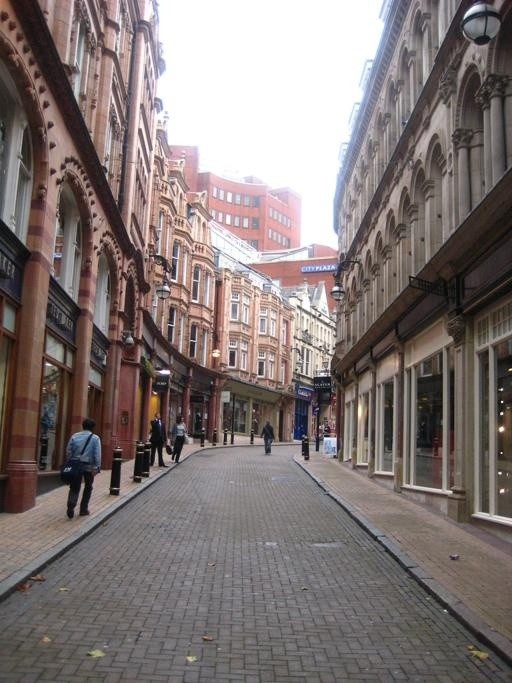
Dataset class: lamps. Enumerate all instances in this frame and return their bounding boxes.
[457,0,503,47]
[211,334,221,357]
[153,265,173,299]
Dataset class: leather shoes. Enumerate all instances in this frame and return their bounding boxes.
[158,464,170,467]
[67,509,90,518]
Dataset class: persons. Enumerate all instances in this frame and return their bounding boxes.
[260,419,276,454]
[317,418,336,439]
[64,417,102,517]
[171,415,191,462]
[150,411,169,467]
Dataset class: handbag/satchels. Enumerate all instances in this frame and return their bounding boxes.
[166,445,172,455]
[61,460,81,484]
